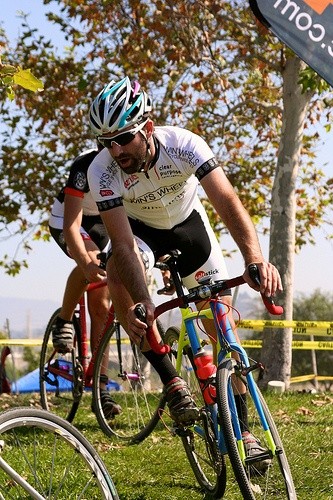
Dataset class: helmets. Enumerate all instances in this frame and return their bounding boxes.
[88,75,152,137]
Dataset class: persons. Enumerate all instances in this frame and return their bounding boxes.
[49,141,126,425]
[95,75,283,478]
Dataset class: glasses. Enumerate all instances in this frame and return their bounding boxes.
[104,116,149,150]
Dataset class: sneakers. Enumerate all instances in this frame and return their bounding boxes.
[91,390,125,415]
[51,317,75,354]
[242,435,271,470]
[161,382,200,422]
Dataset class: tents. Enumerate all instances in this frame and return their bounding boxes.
[7,358,121,392]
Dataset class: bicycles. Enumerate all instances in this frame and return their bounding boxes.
[0,406,120,500]
[38,252,175,446]
[133,247,298,500]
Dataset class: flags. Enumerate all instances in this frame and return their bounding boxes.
[248,0,333,90]
[0,346,12,394]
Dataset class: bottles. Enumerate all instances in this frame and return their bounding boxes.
[194,340,217,404]
